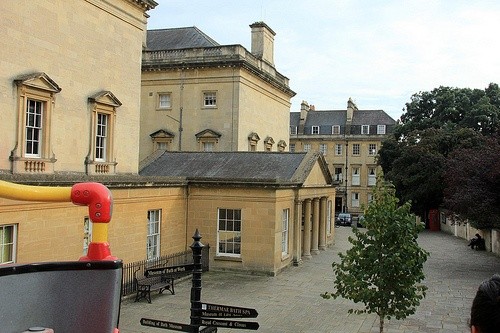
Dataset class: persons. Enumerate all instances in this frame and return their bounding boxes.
[468,232,482,248]
[468,274,500,333]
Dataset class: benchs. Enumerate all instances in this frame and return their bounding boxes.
[134,274,175,304]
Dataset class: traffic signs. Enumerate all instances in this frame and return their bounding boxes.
[139,264,260,333]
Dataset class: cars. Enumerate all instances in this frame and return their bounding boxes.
[357,215,367,228]
[336,214,352,226]
